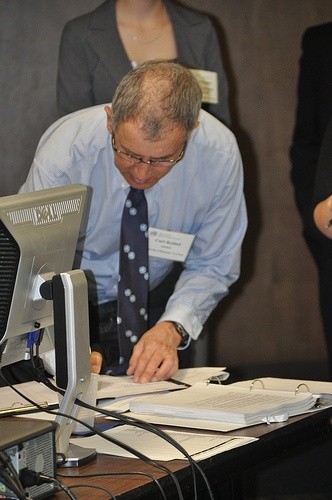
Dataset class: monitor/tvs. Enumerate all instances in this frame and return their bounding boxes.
[0,184,99,468]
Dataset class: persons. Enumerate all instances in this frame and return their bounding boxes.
[15,59,250,385]
[56,0,235,371]
[289,20,332,381]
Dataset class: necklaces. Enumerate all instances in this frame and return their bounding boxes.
[116,12,166,45]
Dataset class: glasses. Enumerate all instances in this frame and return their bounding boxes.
[111,130,188,168]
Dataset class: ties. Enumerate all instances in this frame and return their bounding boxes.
[102,185,150,376]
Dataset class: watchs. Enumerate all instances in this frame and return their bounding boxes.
[172,321,190,346]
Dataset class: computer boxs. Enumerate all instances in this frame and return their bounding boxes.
[0,417,56,500]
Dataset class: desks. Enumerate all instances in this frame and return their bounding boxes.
[14,407,332,500]
